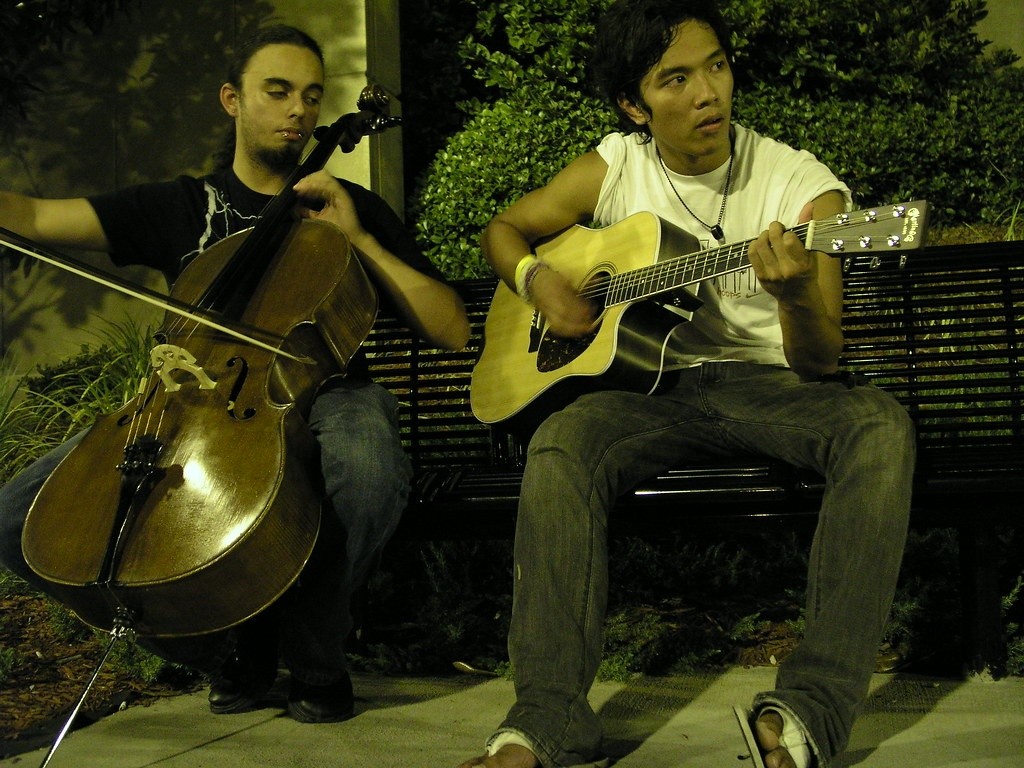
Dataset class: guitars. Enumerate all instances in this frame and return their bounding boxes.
[471,198,931,429]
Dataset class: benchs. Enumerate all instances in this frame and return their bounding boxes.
[362,239,1024,671]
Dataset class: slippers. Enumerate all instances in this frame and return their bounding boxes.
[484,728,537,758]
[732,700,814,768]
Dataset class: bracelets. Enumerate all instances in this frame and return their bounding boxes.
[514,254,548,302]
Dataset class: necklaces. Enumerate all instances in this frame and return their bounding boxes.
[656,142,734,240]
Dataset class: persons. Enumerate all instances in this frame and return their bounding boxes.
[457,0,916,768]
[0,21,471,724]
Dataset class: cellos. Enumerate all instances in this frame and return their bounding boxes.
[6,82,407,768]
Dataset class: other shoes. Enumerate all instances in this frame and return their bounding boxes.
[286,690,339,723]
[208,681,255,714]
[454,653,499,675]
